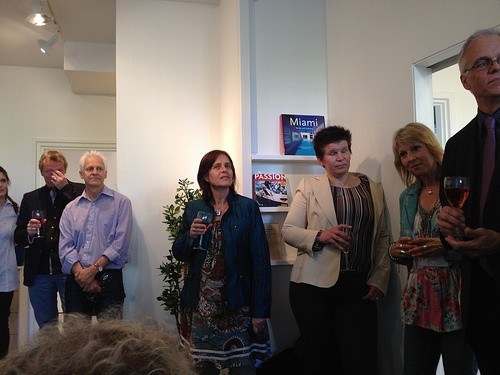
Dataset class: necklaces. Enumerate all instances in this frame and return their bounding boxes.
[328,172,349,187]
[213,204,228,216]
[423,183,436,195]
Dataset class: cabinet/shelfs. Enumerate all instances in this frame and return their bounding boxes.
[251,155,319,266]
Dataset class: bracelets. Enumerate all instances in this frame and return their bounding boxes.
[389,242,400,261]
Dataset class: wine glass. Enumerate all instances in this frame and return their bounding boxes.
[193,211,212,251]
[444,176,470,236]
[32,209,44,239]
[84,271,112,303]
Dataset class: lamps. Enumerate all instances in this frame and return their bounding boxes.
[24,0,54,27]
[37,33,59,56]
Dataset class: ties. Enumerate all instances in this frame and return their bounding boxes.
[479,116,496,227]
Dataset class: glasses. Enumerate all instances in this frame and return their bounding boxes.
[462,56,500,73]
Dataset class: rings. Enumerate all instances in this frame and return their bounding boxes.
[401,250,405,254]
[423,245,428,249]
[400,243,402,247]
[263,326,265,328]
[54,177,57,180]
[376,297,379,301]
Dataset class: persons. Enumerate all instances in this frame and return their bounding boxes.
[437,29,500,375]
[281,125,391,375]
[0,314,195,375]
[265,179,287,194]
[0,166,20,360]
[171,150,272,375]
[388,122,478,375]
[58,150,132,321]
[13,150,85,328]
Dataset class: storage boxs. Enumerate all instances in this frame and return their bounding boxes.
[280,114,325,155]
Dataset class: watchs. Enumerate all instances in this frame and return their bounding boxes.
[93,262,103,272]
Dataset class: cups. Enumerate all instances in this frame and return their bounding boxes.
[404,228,432,256]
[335,228,350,251]
